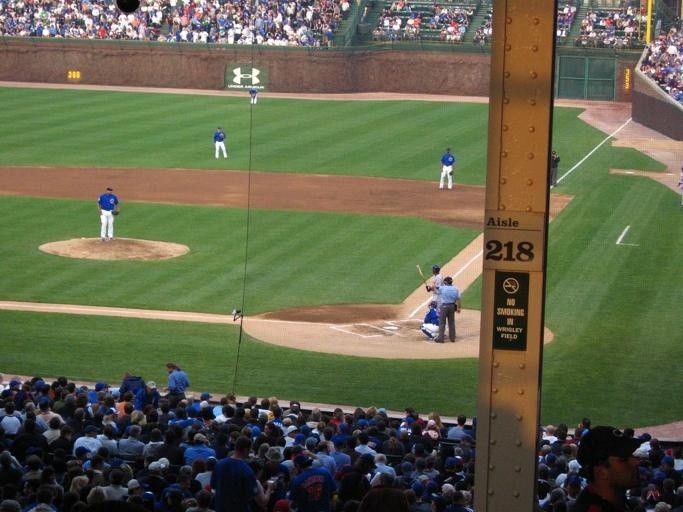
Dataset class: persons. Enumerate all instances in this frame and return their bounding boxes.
[97,186,120,242]
[551,150,560,186]
[437,147,453,191]
[1,0,683,111]
[0,360,683,512]
[213,126,228,160]
[418,265,461,343]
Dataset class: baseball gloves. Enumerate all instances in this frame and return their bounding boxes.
[219,133,225,138]
[112,210,118,215]
[450,171,453,175]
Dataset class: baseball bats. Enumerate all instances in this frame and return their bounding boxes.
[416,265,431,293]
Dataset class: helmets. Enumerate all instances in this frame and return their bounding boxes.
[433,265,440,270]
[444,277,452,284]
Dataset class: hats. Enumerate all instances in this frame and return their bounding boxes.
[127,478,148,490]
[84,425,101,433]
[265,447,284,463]
[201,393,213,400]
[429,301,436,306]
[76,446,90,455]
[148,428,163,442]
[576,427,646,468]
[9,381,21,387]
[147,381,157,390]
[105,408,120,415]
